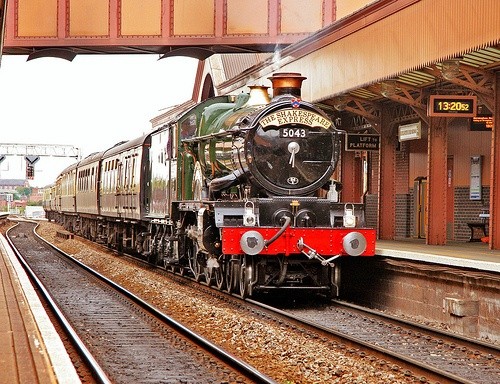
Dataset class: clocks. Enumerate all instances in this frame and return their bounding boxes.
[426,94,478,118]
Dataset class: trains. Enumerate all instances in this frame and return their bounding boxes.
[41,71,376,302]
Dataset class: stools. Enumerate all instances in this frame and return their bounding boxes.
[465,222,489,242]
